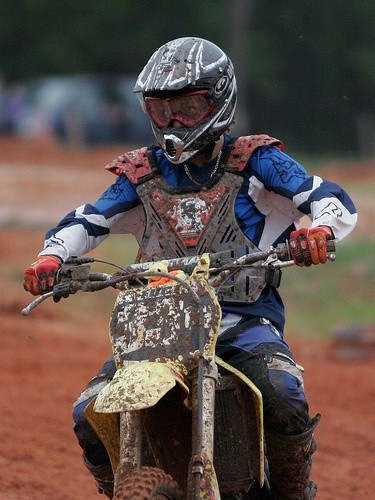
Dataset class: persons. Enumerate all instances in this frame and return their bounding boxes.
[24,35,359,500]
[1,77,153,147]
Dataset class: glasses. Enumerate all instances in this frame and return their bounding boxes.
[144,90,216,129]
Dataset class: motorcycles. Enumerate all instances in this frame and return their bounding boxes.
[21,240,336,500]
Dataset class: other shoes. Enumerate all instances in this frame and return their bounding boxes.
[265,411,323,500]
[82,451,114,500]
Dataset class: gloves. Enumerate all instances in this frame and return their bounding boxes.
[23,255,63,297]
[288,225,336,268]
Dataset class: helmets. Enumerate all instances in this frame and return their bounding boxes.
[133,35,240,168]
